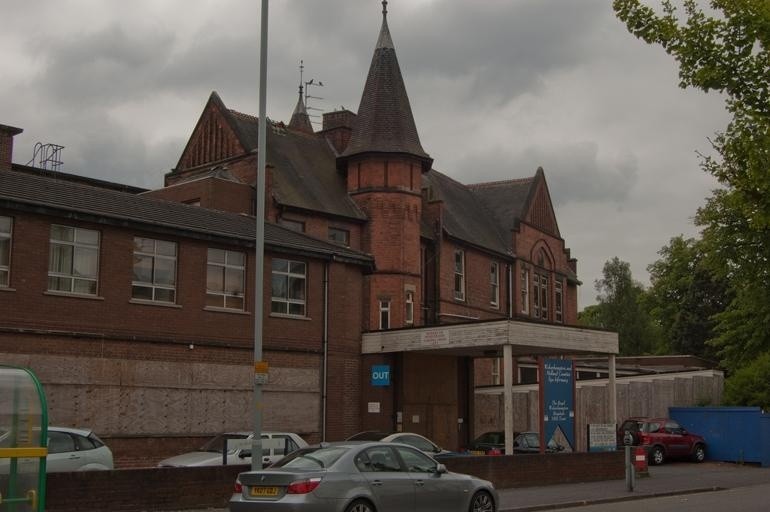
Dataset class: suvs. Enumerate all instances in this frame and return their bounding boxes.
[618,416,707,466]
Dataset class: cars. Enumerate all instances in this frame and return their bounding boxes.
[0,426,114,472]
[229,441,500,511]
[458,431,565,455]
[157,431,309,468]
[345,430,452,458]
